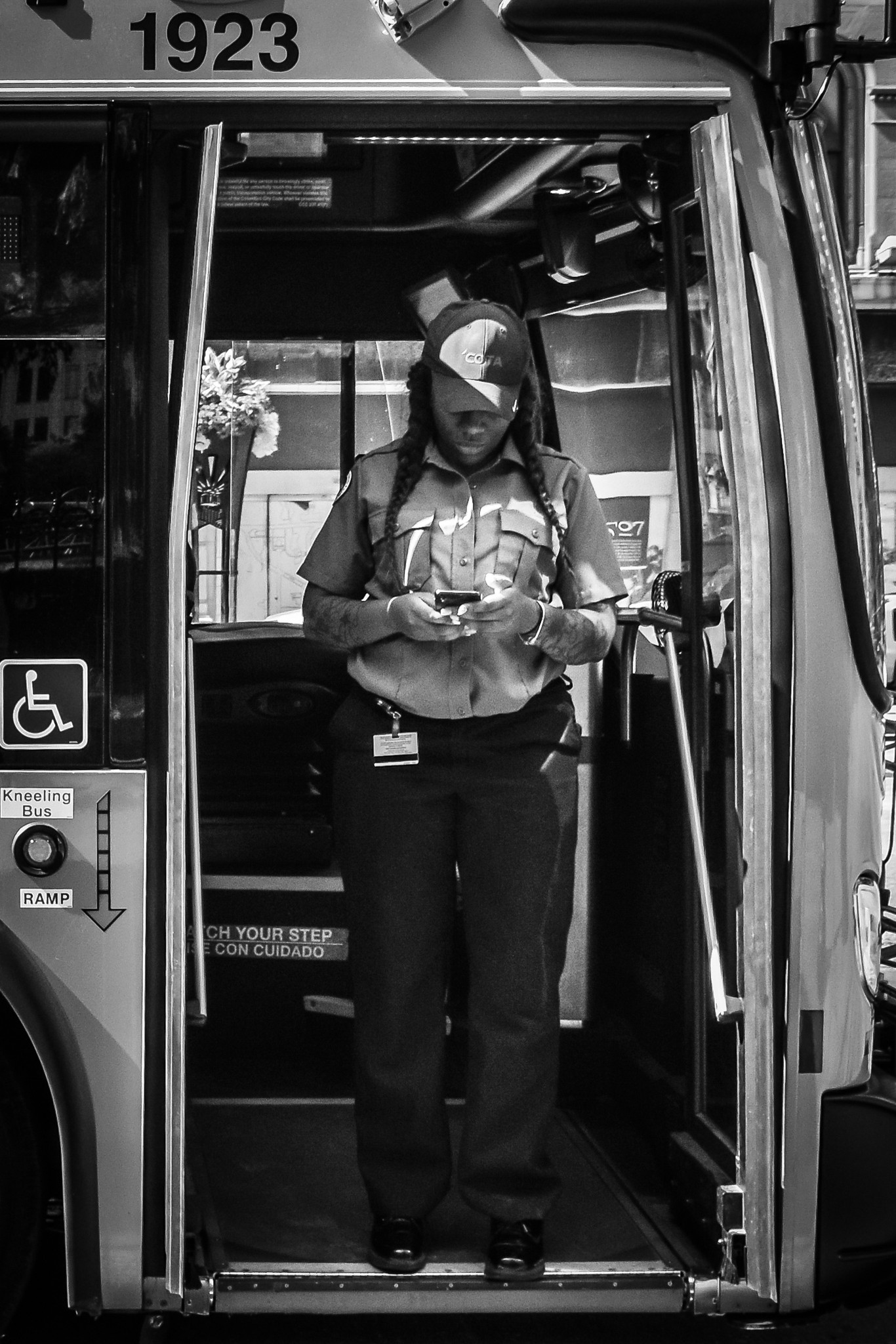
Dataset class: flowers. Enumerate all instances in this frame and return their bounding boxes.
[196,347,283,458]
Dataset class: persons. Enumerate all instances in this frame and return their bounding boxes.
[300,297,629,1286]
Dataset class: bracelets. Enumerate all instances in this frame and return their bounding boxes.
[386,596,400,621]
[517,601,546,647]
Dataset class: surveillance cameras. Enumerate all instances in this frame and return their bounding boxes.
[582,165,620,192]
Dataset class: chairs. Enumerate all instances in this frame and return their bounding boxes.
[187,548,345,681]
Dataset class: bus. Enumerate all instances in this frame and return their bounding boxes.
[0,0,895,1344]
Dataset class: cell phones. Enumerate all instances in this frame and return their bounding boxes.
[434,589,482,612]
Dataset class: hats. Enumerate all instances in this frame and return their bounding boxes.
[420,299,531,421]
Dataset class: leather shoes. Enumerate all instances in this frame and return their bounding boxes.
[371,1213,427,1274]
[485,1216,547,1281]
